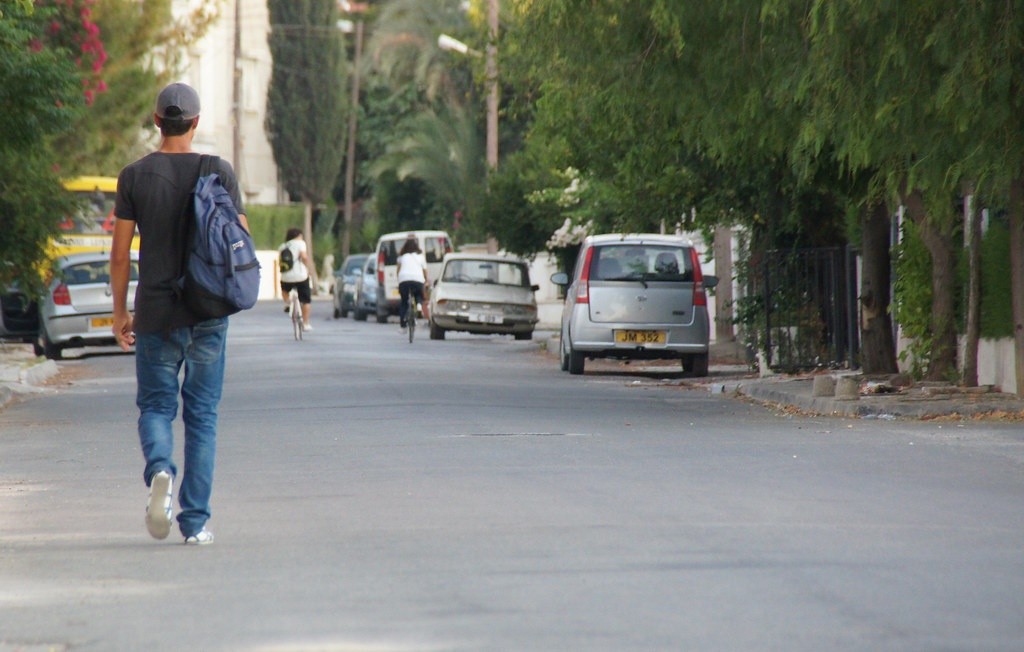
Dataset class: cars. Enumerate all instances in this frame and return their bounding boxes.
[332,253,368,319]
[353,253,378,320]
[427,252,540,341]
[34,250,139,360]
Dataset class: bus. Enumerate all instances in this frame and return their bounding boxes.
[32,175,141,287]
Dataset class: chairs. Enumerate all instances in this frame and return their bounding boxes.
[595,250,680,278]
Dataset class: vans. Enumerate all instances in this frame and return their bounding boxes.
[375,229,455,324]
[549,234,720,376]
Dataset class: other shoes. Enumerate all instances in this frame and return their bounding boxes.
[399,327,406,334]
[303,324,311,331]
[416,303,423,320]
[284,304,290,312]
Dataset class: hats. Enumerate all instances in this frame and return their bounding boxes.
[156,83,200,120]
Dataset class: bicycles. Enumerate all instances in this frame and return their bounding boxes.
[287,288,305,342]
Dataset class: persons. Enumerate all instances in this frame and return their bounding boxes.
[279,227,313,330]
[396,239,431,328]
[110,83,250,544]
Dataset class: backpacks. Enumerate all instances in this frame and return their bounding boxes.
[166,155,260,334]
[280,242,293,274]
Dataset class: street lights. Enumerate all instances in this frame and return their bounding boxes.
[336,0,367,255]
[439,0,498,170]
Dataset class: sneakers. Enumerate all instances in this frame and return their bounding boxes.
[185,527,214,545]
[145,471,175,539]
